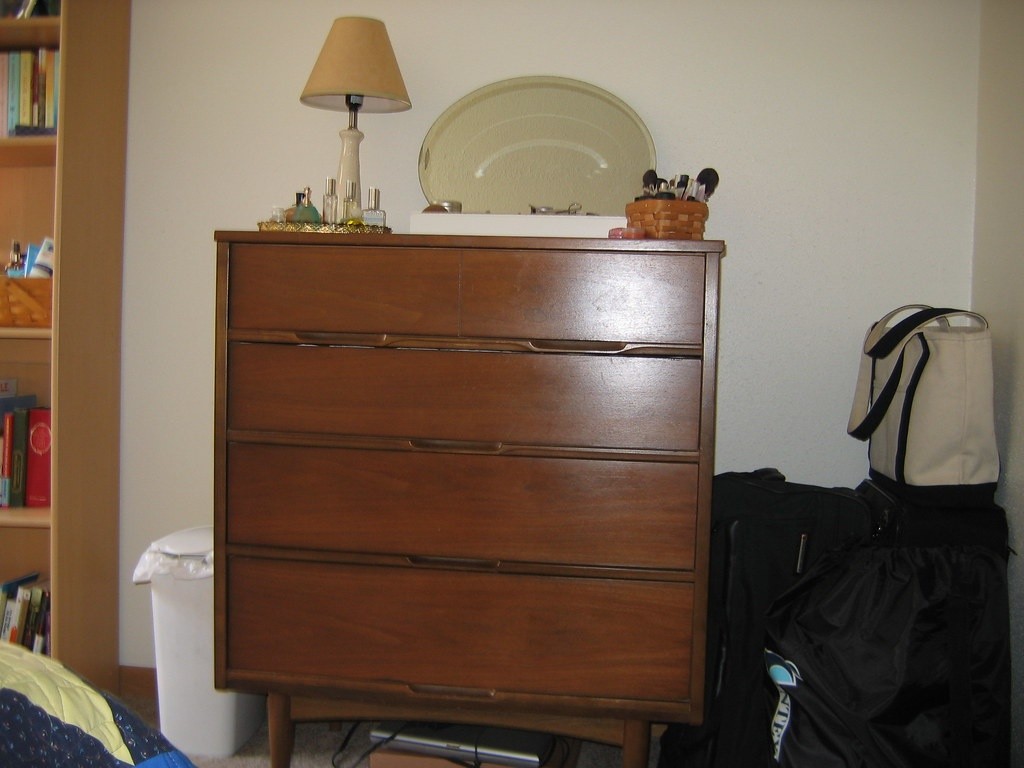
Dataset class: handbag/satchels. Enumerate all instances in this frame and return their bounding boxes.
[658,468,1014,767]
[846,303,1001,503]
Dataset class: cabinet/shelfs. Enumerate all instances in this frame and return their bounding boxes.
[212,229,726,768]
[0,0,133,698]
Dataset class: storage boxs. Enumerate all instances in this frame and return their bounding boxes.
[409,212,628,238]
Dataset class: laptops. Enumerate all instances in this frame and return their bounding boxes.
[368,722,556,768]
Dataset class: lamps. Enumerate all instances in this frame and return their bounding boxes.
[301,17,412,222]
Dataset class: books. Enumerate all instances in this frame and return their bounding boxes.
[0,0,61,20]
[0,572,51,658]
[0,47,59,137]
[0,394,52,510]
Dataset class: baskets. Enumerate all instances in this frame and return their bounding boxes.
[625,199,708,242]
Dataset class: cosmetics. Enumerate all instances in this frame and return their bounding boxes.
[343,182,358,220]
[642,168,720,203]
[608,227,645,238]
[28,237,54,278]
[285,192,304,223]
[430,200,462,214]
[294,188,320,223]
[322,178,338,224]
[269,206,286,223]
[361,187,385,227]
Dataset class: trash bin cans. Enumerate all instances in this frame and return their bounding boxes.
[145,527,268,758]
